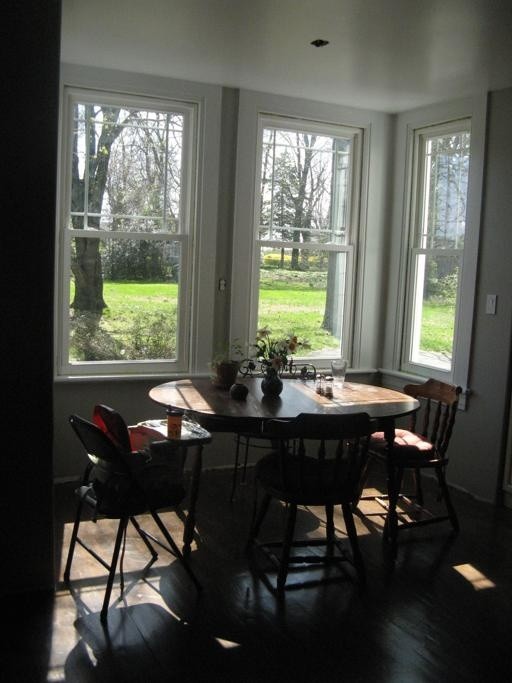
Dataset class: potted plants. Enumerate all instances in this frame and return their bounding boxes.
[205,336,240,388]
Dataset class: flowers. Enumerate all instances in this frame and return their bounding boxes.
[233,324,313,378]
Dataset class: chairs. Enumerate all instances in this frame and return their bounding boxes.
[93,402,213,474]
[349,379,460,573]
[250,411,373,621]
[64,411,205,621]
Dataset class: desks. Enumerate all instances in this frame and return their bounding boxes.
[146,381,421,544]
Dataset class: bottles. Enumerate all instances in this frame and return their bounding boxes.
[315,375,334,399]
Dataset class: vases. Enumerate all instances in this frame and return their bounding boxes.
[261,370,284,398]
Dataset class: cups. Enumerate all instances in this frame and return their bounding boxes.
[165,409,184,438]
[331,359,348,386]
